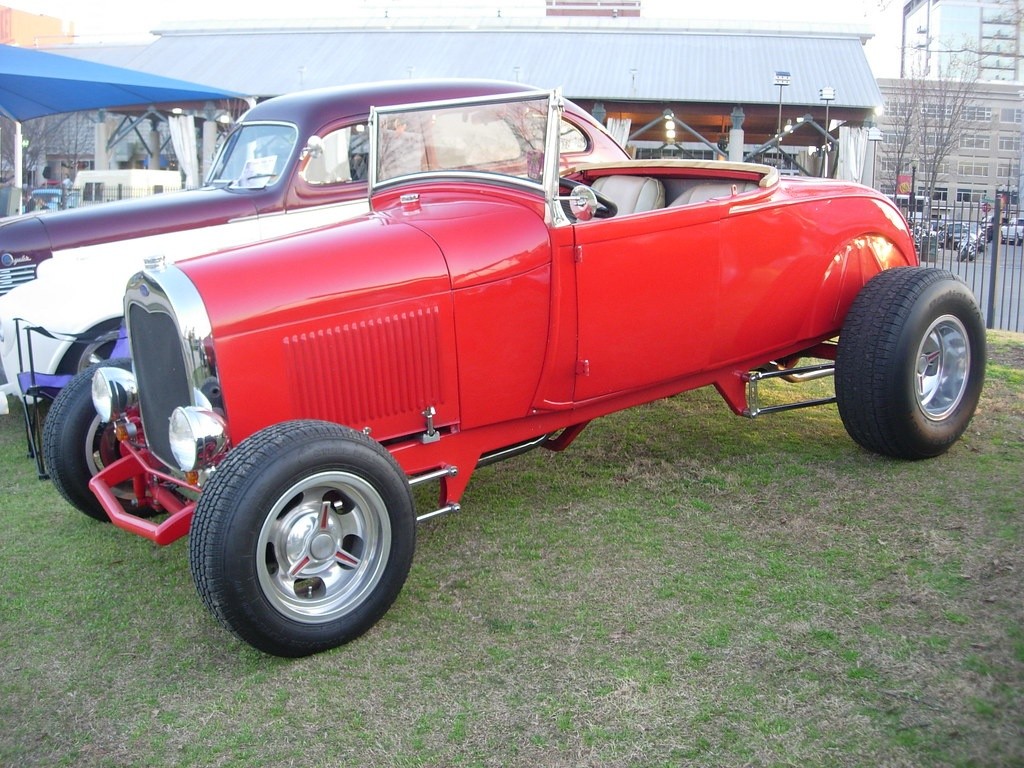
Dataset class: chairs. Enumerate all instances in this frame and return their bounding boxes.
[576,175,666,223]
[667,182,760,207]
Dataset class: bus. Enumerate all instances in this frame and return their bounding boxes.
[883,194,931,225]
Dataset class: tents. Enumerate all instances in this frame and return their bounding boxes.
[0,44,257,216]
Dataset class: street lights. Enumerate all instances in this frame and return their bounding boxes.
[776,71,791,174]
[818,86,834,177]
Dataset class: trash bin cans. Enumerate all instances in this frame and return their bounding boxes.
[921,237,938,262]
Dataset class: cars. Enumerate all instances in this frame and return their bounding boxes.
[931,210,1024,253]
[0,80,638,408]
[42,85,987,660]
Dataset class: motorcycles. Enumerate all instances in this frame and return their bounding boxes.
[957,220,990,263]
[908,217,928,252]
